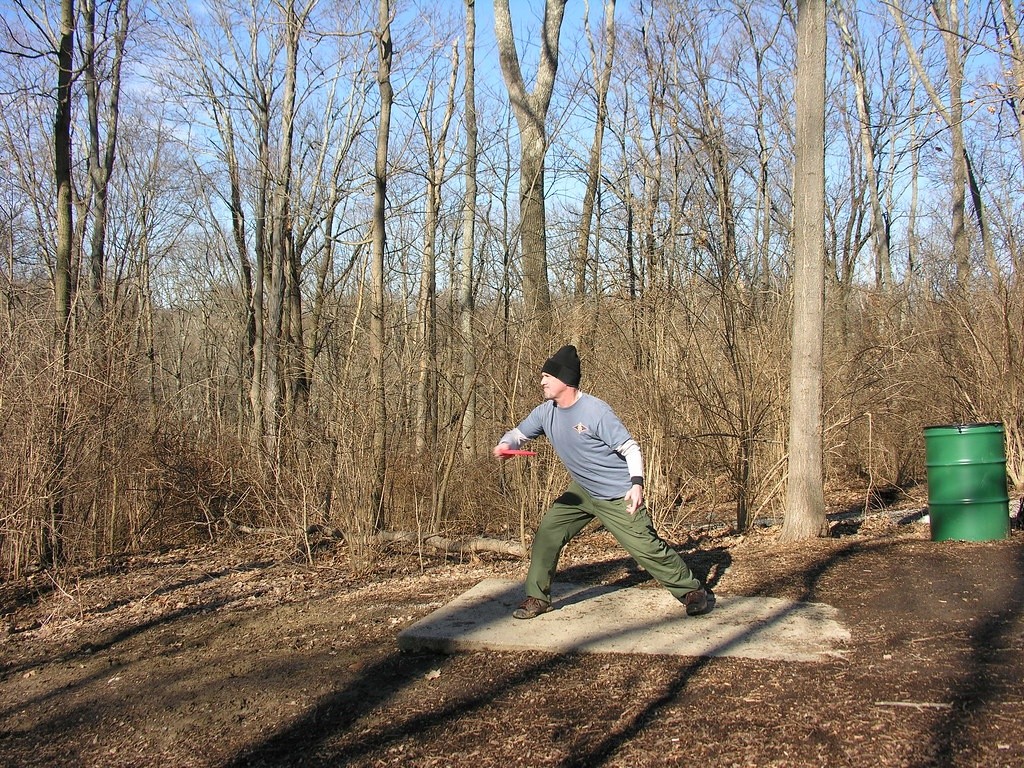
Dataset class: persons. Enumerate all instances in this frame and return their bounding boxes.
[493,344,708,619]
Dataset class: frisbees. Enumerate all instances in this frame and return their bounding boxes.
[498,449,538,457]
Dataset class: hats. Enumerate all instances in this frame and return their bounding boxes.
[541,345,581,388]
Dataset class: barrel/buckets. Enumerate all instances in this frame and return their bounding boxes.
[923,421,1011,542]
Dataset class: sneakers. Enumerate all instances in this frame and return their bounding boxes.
[685,588,708,616]
[513,597,553,619]
